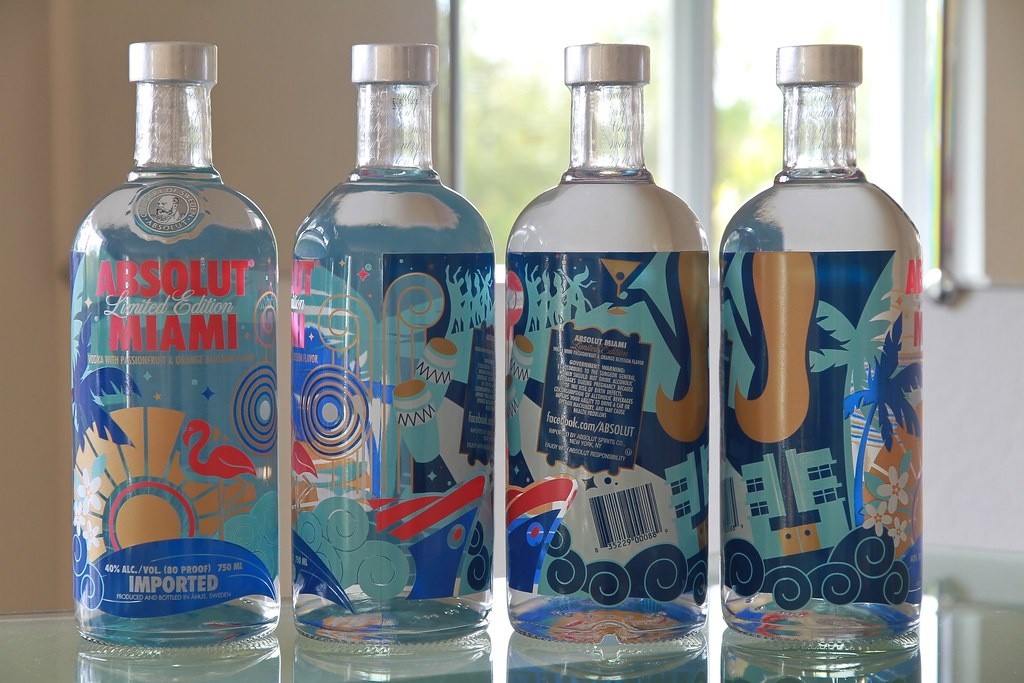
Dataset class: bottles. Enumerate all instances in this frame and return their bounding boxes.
[720,44,925,654]
[502,44,709,662]
[70,42,281,661]
[291,43,496,667]
